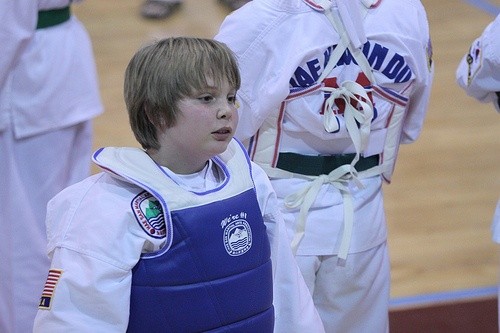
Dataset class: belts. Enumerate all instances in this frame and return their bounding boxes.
[36,6,71,29]
[275,152,380,176]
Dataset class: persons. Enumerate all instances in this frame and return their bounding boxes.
[0,0,103,333]
[36,38,322,333]
[213,0,433,333]
[455,10,500,246]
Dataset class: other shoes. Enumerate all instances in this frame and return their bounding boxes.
[220,0,253,10]
[141,0,183,19]
